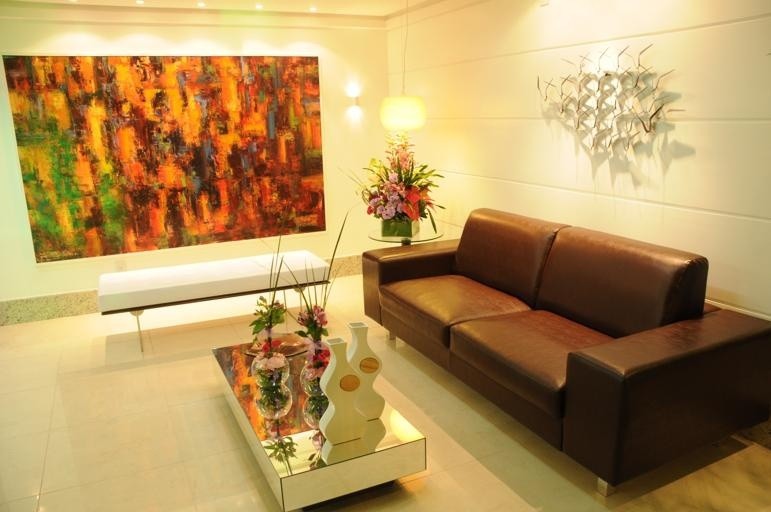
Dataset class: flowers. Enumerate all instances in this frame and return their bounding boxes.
[259,420,298,476]
[248,200,291,358]
[306,431,327,470]
[282,205,351,368]
[360,136,444,232]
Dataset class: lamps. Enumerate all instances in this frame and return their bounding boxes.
[376,1,429,129]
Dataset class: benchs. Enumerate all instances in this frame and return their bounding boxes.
[97,251,330,323]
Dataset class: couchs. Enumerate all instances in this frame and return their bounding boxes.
[362,207,771,497]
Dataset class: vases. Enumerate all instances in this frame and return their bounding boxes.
[254,354,291,387]
[255,386,292,419]
[302,362,334,398]
[381,220,420,238]
[302,396,329,430]
